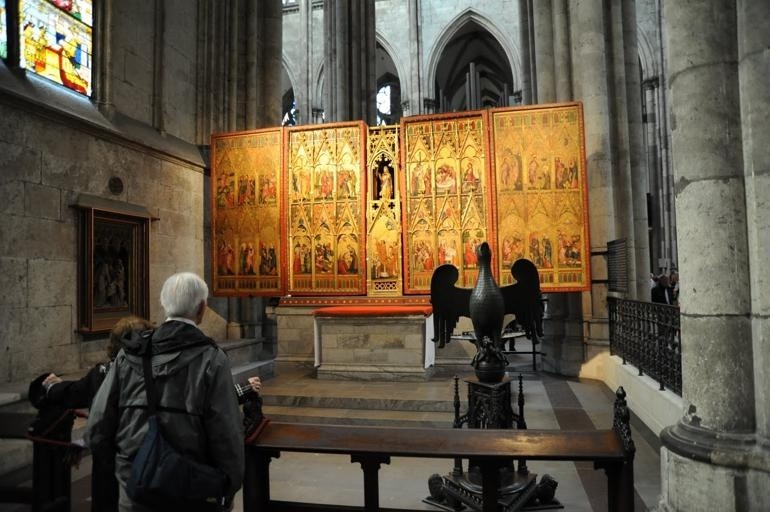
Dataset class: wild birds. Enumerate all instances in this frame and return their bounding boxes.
[430,242,545,349]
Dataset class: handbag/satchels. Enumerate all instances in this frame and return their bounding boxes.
[125,417,227,512]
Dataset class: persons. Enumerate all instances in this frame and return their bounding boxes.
[41,315,261,512]
[650,270,679,306]
[83,271,245,512]
